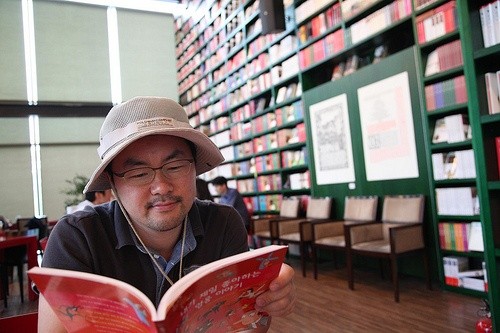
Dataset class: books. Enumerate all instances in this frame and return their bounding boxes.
[172,0,499,294]
[26,244,289,333]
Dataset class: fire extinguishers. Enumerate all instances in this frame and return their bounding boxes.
[475,297,498,333]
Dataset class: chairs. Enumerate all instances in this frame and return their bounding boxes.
[347,194,430,303]
[311,196,378,290]
[16,216,48,263]
[276,196,332,277]
[251,200,300,260]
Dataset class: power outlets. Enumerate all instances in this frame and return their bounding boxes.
[348,183,356,190]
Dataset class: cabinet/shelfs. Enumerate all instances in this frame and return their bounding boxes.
[173,0,500,333]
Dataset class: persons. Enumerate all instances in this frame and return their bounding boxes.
[71,186,113,211]
[211,177,250,237]
[37,95,297,333]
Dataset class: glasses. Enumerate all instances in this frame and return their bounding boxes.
[104,155,196,186]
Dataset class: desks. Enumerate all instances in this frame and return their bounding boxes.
[0,234,40,302]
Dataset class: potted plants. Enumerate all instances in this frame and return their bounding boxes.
[60,176,90,215]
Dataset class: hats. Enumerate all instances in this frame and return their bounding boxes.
[82,96,225,194]
